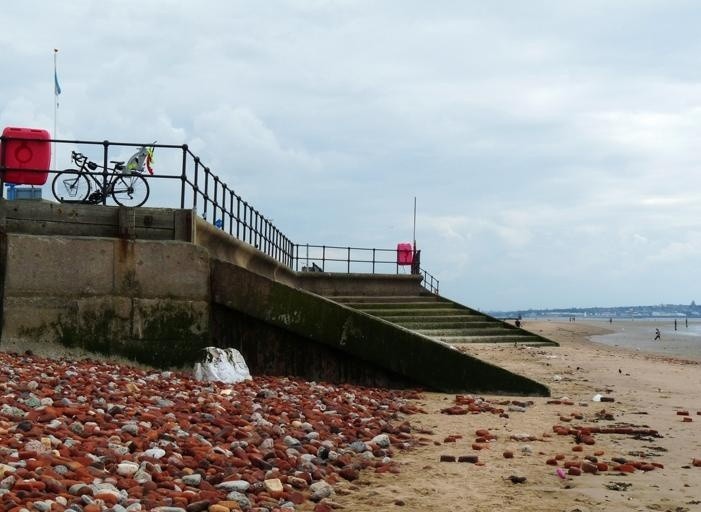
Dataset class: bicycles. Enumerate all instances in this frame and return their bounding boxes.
[52,151,150,207]
